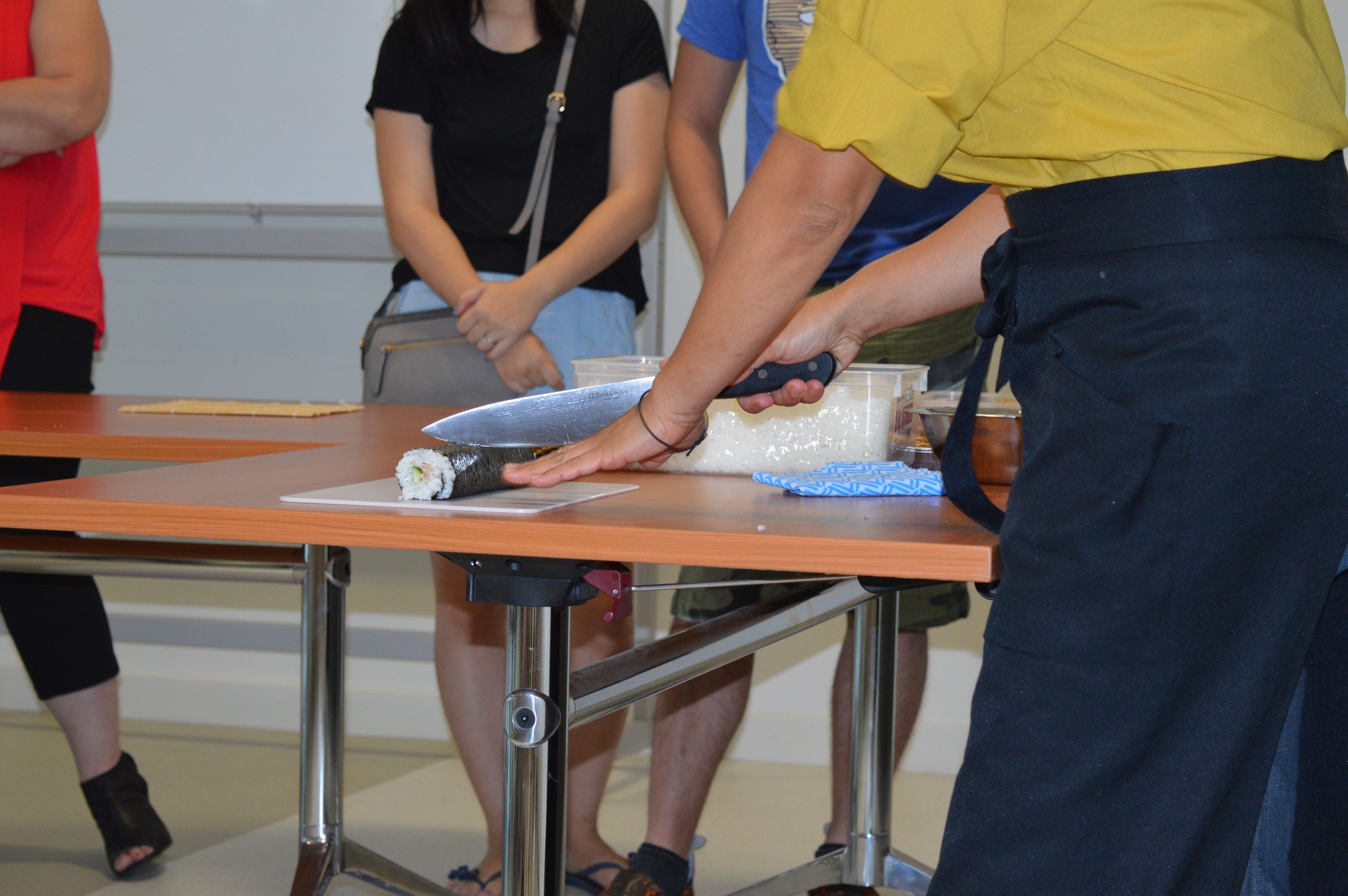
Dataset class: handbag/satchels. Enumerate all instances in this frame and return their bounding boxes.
[363,306,521,408]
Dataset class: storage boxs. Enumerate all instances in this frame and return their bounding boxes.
[573,356,930,472]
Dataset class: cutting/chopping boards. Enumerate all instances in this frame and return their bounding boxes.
[279,476,639,515]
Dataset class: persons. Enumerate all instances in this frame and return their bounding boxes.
[501,0,1348,896]
[0,0,171,879]
[616,0,990,896]
[364,0,675,896]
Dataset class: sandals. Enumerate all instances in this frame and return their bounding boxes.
[79,752,173,876]
[447,865,503,896]
[565,862,624,896]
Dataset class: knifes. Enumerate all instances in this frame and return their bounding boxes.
[421,351,838,448]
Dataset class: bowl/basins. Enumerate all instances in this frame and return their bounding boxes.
[904,406,1022,486]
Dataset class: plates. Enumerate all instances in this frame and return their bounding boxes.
[890,428,935,454]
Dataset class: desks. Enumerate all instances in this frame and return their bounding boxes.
[0,389,1014,896]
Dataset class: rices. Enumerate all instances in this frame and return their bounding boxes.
[621,386,915,476]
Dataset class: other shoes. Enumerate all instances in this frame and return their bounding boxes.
[599,834,707,896]
[808,823,881,896]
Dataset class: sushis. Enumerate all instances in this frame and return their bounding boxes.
[395,442,539,501]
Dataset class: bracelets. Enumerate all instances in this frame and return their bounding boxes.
[638,389,709,456]
